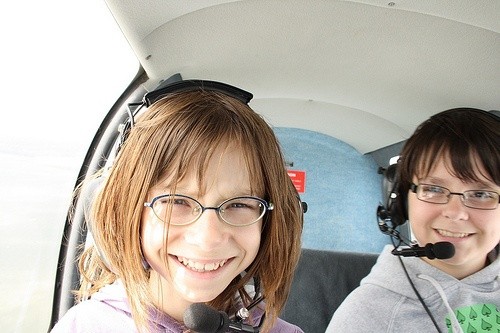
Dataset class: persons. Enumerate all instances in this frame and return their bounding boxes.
[323,107,500,333]
[48,80,305,333]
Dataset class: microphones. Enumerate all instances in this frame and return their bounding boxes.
[394,242,455,260]
[183,303,260,333]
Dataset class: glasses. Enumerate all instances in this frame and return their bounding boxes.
[144,194,275,227]
[409,183,500,210]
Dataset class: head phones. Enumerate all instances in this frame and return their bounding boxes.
[101,73,308,328]
[378,108,500,251]
